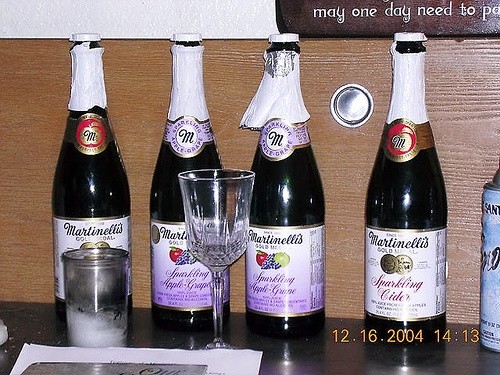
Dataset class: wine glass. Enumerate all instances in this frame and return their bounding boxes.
[178,169,256,349]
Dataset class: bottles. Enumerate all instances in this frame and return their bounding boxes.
[240,33,327,337]
[52,34,133,319]
[363,32,447,345]
[149,34,229,331]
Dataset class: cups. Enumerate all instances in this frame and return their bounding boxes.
[62,249,129,347]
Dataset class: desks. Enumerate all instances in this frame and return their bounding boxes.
[0,299,500,374]
